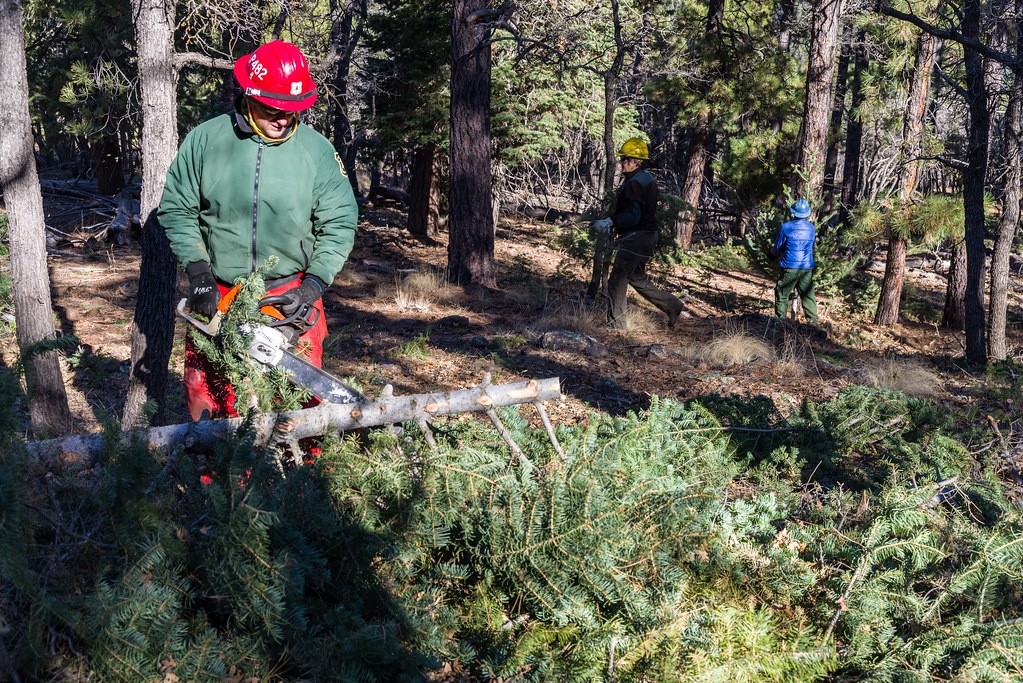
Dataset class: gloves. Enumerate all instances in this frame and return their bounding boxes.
[185,260,220,319]
[594,216,614,235]
[274,273,326,322]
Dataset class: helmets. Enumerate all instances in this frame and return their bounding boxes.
[790,199,812,219]
[234,41,318,112]
[615,137,650,161]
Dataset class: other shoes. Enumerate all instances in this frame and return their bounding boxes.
[602,320,627,329]
[669,302,684,327]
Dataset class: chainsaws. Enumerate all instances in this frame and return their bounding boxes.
[176,293,371,403]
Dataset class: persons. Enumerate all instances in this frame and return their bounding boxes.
[157,42,359,484]
[595,137,684,333]
[774,198,822,326]
